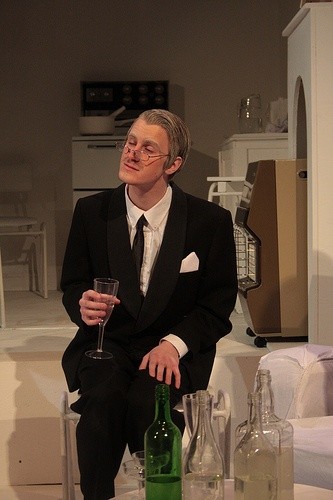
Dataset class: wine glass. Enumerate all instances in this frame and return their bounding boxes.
[84,278,119,360]
[183,393,215,490]
[123,451,169,500]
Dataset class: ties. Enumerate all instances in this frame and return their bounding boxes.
[130,214,149,289]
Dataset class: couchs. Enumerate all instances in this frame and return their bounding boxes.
[243,344,333,491]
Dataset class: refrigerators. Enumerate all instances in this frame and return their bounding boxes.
[70,136,126,209]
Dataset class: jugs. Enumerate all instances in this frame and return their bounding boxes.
[239,93,262,134]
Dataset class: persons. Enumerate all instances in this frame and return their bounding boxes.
[61,109,238,500]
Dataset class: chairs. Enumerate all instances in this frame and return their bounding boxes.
[0,167,48,300]
[60,384,232,500]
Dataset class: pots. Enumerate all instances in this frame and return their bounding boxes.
[77,116,137,135]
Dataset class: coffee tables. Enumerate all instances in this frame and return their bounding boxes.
[112,473,333,500]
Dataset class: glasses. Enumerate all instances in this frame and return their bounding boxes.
[116,143,170,161]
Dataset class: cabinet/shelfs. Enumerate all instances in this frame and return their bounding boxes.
[218,132,289,224]
[72,134,129,211]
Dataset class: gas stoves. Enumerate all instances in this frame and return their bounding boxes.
[71,80,170,142]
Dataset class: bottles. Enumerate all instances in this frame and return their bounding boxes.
[143,384,184,500]
[235,369,294,500]
[181,390,225,500]
[233,392,277,500]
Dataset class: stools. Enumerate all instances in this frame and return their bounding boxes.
[207,177,245,203]
